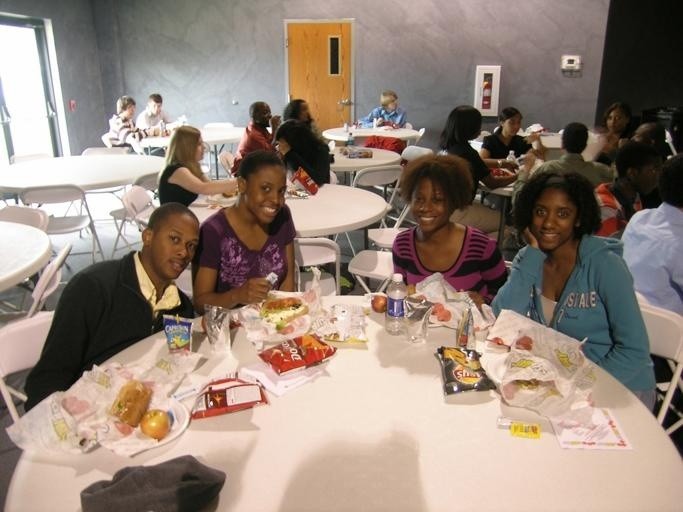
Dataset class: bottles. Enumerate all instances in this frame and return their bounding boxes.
[507,150,515,161]
[385,273,407,336]
[347,133,354,148]
[160,120,166,137]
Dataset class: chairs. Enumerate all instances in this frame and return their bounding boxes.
[122,185,157,233]
[348,249,394,293]
[20,184,105,269]
[406,122,413,145]
[1,207,71,273]
[368,204,413,248]
[635,291,683,391]
[639,302,683,434]
[293,237,342,294]
[416,128,426,144]
[79,147,128,239]
[1,312,56,422]
[204,122,234,180]
[28,244,73,320]
[334,165,402,256]
[11,152,90,236]
[373,147,434,214]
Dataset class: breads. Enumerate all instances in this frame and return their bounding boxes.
[110,379,152,427]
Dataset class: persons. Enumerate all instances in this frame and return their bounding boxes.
[631,121,666,145]
[157,125,237,209]
[620,152,683,318]
[355,91,407,152]
[191,151,297,311]
[274,119,330,185]
[230,101,279,177]
[108,95,165,159]
[23,203,200,411]
[439,105,519,234]
[478,106,545,169]
[392,155,508,308]
[592,102,632,160]
[503,122,614,250]
[283,99,335,163]
[135,93,163,130]
[592,140,661,239]
[490,170,657,410]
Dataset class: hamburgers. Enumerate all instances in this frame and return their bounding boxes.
[262,297,309,324]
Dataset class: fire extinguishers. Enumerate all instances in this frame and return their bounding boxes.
[482,77,492,109]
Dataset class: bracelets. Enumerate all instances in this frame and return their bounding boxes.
[496,158,503,168]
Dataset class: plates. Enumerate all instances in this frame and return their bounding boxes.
[97,391,191,457]
[249,310,311,341]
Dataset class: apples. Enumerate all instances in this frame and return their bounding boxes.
[140,410,170,440]
[371,296,387,313]
